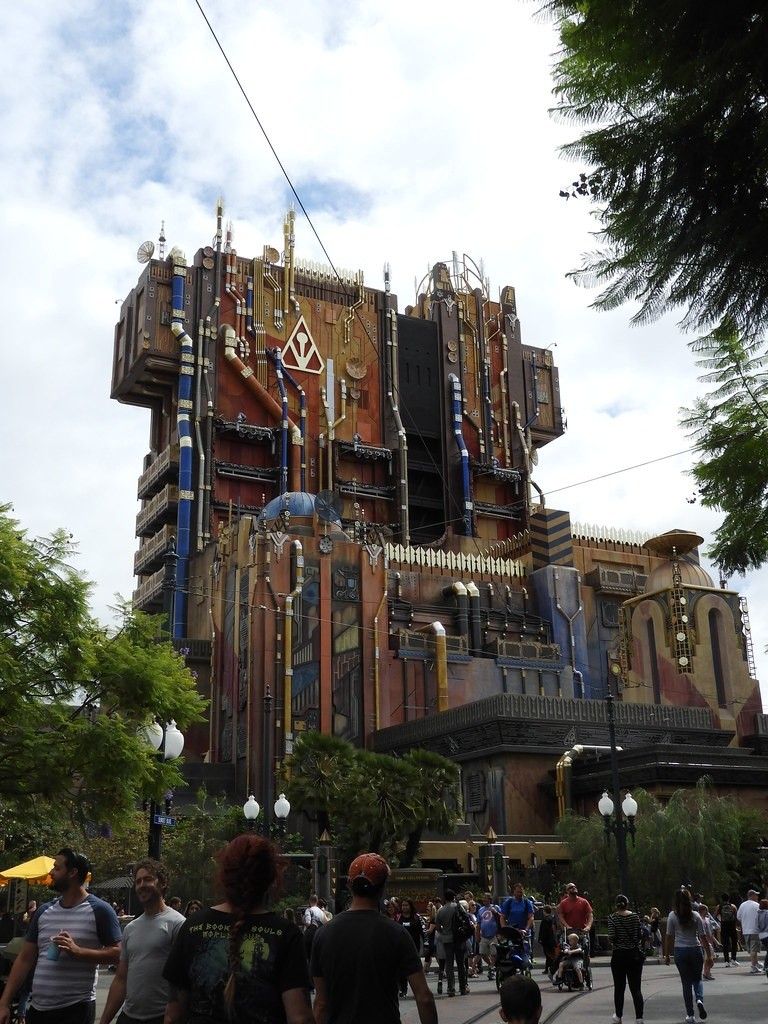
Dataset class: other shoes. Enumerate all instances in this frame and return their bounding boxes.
[487,972,495,981]
[579,982,584,988]
[423,962,431,973]
[750,966,766,975]
[553,978,563,985]
[756,963,763,969]
[725,962,731,967]
[469,972,479,978]
[542,970,548,973]
[460,987,470,994]
[613,1014,622,1024]
[685,1015,695,1023]
[635,1019,644,1024]
[437,981,443,994]
[449,990,455,997]
[399,989,408,998]
[731,960,739,967]
[697,1000,707,1019]
[702,973,715,981]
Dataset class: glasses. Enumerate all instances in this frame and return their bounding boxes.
[567,885,576,890]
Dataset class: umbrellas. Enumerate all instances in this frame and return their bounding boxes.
[0,854,92,905]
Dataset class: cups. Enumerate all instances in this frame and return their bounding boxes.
[46,937,61,961]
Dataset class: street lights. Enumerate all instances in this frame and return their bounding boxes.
[134,714,185,862]
[241,789,290,908]
[599,663,638,909]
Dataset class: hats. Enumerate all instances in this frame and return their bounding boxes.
[349,852,390,893]
[747,890,760,896]
[694,893,704,899]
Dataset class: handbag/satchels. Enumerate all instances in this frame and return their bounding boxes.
[634,946,646,961]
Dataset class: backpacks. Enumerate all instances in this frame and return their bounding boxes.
[452,903,472,943]
[720,902,735,922]
[302,910,322,952]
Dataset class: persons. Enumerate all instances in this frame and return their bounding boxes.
[162,833,315,1024]
[665,889,707,1023]
[607,895,645,1024]
[0,879,768,996]
[309,852,438,1024]
[99,859,188,1024]
[498,974,543,1024]
[0,848,122,1024]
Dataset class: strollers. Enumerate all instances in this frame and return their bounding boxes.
[554,926,593,992]
[492,927,534,991]
